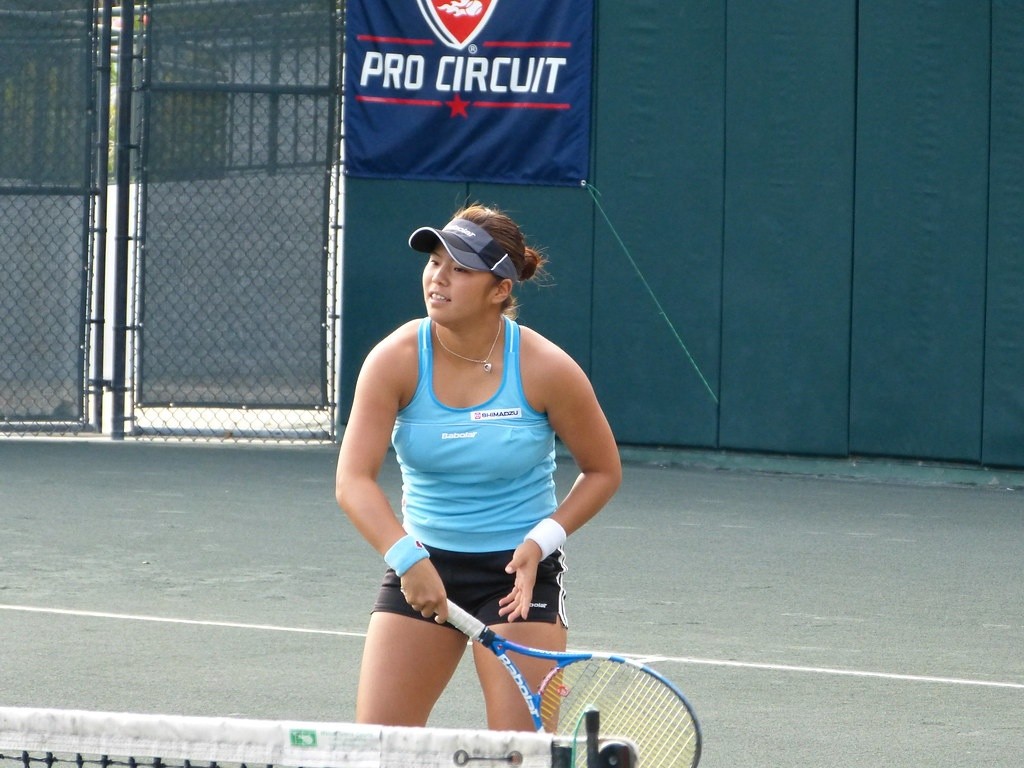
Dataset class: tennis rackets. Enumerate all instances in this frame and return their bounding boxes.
[400,585,703,768]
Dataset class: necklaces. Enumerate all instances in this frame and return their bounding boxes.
[433,314,502,373]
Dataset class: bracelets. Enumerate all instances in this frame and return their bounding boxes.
[524,518,568,563]
[384,532,430,579]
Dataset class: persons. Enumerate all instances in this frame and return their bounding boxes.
[332,203,621,734]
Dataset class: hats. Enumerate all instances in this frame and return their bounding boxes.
[408,216,518,280]
